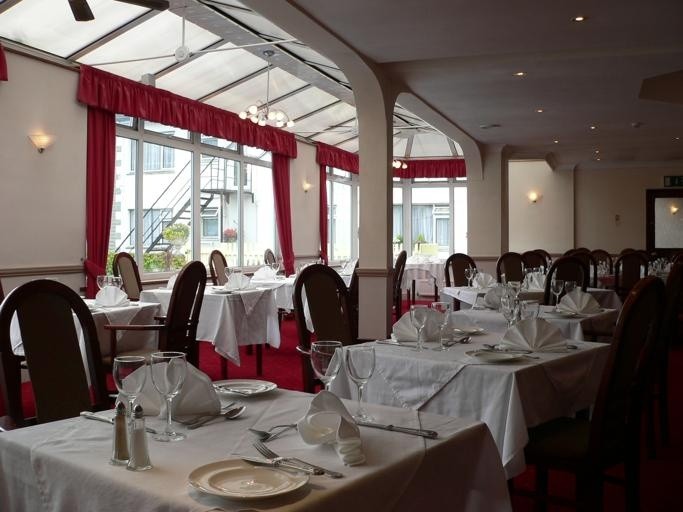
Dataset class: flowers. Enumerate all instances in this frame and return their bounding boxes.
[222,228,237,238]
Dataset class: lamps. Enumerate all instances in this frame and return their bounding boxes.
[240,49,297,129]
[26,134,60,153]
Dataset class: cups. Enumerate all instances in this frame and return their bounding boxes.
[498,297,538,326]
[96,256,346,292]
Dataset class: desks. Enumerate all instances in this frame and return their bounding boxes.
[401,255,452,304]
[327,332,610,477]
[0,383,513,512]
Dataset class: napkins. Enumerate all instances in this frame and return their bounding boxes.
[391,304,453,343]
[497,315,568,355]
[296,389,367,469]
[115,360,223,417]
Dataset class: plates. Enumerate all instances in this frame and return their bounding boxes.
[212,378,277,397]
[463,349,524,362]
[185,460,311,501]
[462,327,486,335]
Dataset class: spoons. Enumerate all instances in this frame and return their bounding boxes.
[174,405,247,426]
[247,422,297,440]
[78,411,183,442]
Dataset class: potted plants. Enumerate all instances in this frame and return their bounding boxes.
[163,222,190,249]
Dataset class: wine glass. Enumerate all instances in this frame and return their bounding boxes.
[309,340,343,391]
[411,302,451,353]
[150,352,186,444]
[462,253,668,312]
[112,356,147,433]
[345,345,378,424]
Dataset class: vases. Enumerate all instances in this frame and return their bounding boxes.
[223,236,236,243]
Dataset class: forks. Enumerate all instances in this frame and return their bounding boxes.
[250,439,345,480]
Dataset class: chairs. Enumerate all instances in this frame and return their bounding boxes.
[439,250,683,343]
[528,274,669,510]
[291,261,357,391]
[111,249,409,379]
[0,278,111,428]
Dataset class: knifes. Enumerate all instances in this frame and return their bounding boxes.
[241,454,322,475]
[354,420,438,439]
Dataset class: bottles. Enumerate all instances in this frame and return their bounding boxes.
[128,403,153,472]
[109,401,131,467]
